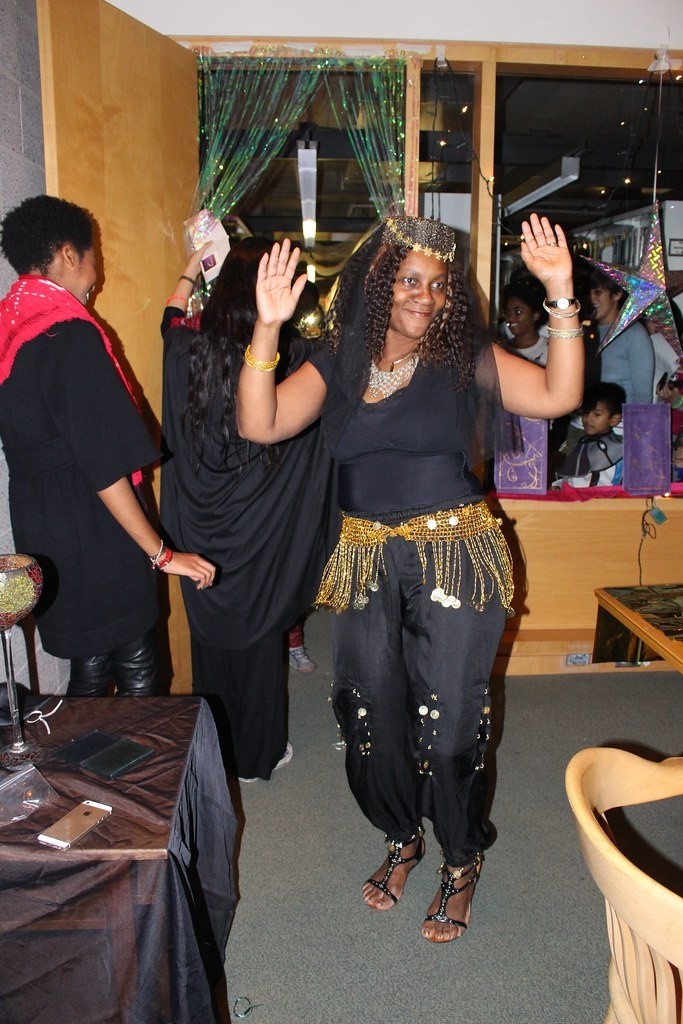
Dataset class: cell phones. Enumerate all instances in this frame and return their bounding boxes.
[37,800,113,851]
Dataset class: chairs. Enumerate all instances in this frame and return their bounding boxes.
[563,748,683,1024]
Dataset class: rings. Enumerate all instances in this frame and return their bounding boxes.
[546,242,556,246]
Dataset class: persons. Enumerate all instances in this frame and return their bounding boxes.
[490,256,683,491]
[157,234,343,780]
[1,195,218,698]
[232,213,587,943]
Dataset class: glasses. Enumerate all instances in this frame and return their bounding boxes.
[501,307,531,319]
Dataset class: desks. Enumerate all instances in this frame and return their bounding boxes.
[2,683,240,1024]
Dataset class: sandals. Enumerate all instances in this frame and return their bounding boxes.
[421,851,485,943]
[362,828,427,910]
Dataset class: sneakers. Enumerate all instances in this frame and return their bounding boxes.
[288,646,316,673]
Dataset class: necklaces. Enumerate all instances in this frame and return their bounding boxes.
[380,350,413,372]
[367,356,420,400]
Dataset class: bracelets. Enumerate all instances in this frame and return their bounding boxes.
[178,276,196,285]
[149,545,166,560]
[149,538,163,570]
[157,547,173,568]
[166,295,189,308]
[243,345,280,371]
